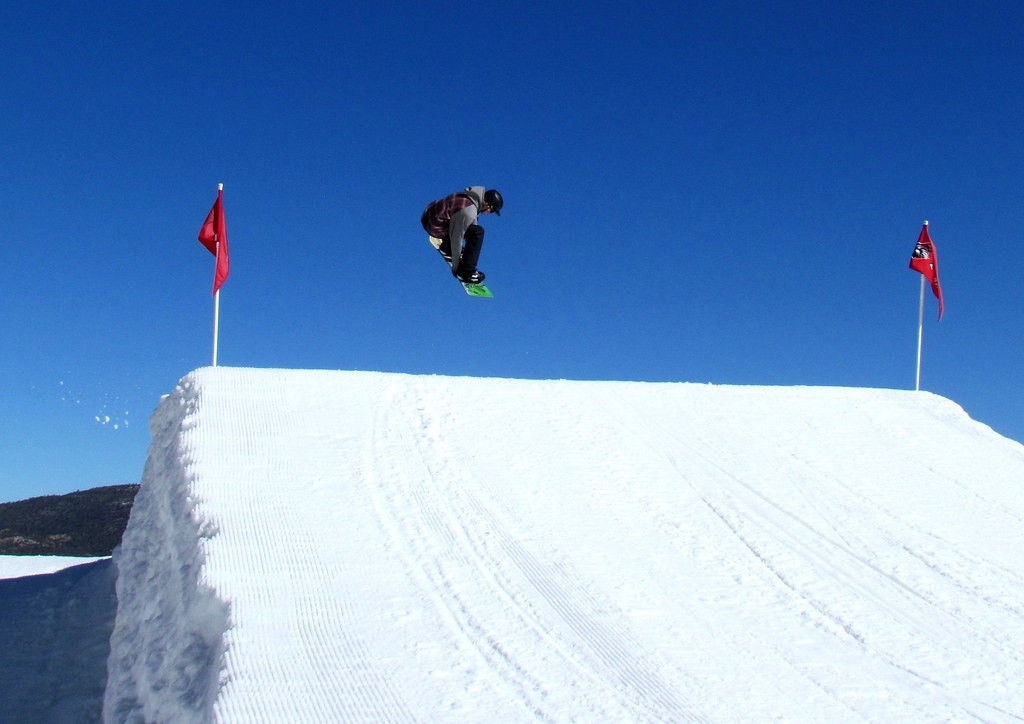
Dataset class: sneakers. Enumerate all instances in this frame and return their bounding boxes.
[457,271,485,283]
[439,244,465,255]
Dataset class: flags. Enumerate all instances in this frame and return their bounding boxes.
[198,190,231,297]
[910,225,944,322]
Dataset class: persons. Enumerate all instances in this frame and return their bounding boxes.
[421,185,503,284]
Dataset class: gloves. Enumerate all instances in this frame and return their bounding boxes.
[452,269,458,278]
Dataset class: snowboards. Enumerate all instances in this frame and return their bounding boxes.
[429,235,494,298]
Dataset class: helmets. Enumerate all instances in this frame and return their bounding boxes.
[484,190,504,216]
